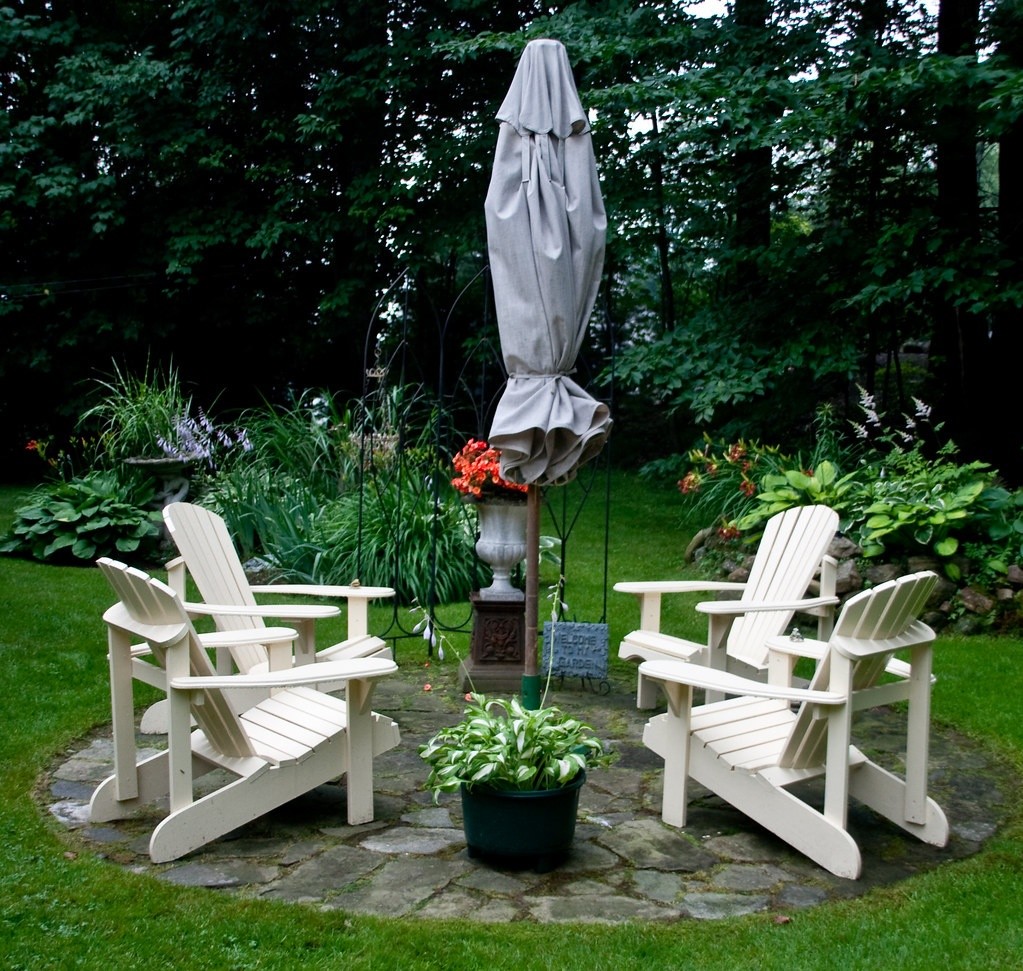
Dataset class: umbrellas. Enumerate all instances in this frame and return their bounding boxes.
[483,36,615,712]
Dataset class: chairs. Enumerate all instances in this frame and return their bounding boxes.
[140,501,397,737]
[638,569,949,881]
[613,504,845,717]
[84,557,402,862]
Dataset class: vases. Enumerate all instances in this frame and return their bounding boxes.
[474,500,529,603]
[460,770,588,871]
[124,452,207,552]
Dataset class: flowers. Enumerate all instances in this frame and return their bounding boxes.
[155,407,255,462]
[454,439,529,501]
[409,576,616,807]
